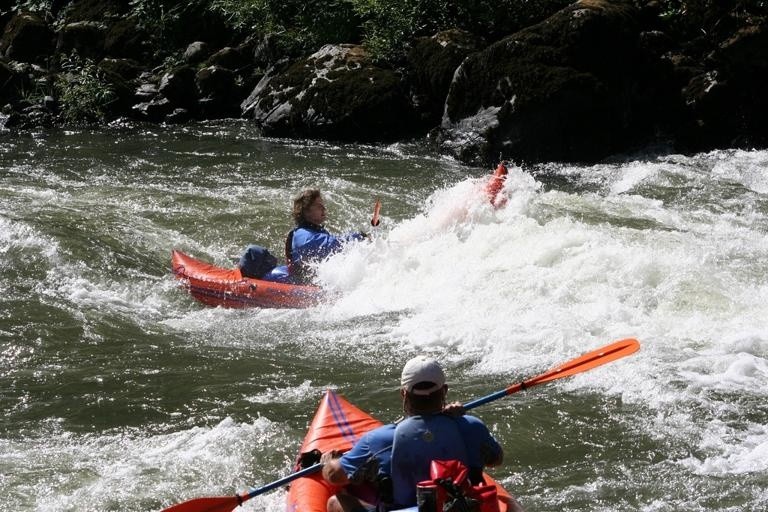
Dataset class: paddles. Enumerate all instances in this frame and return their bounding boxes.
[163,340,640,512]
[370,197,381,232]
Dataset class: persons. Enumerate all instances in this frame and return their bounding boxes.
[319,354,504,512]
[284,188,381,285]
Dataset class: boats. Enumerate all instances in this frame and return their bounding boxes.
[170,159,508,310]
[284,388,524,512]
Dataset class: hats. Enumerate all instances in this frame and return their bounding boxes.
[400,355,446,396]
